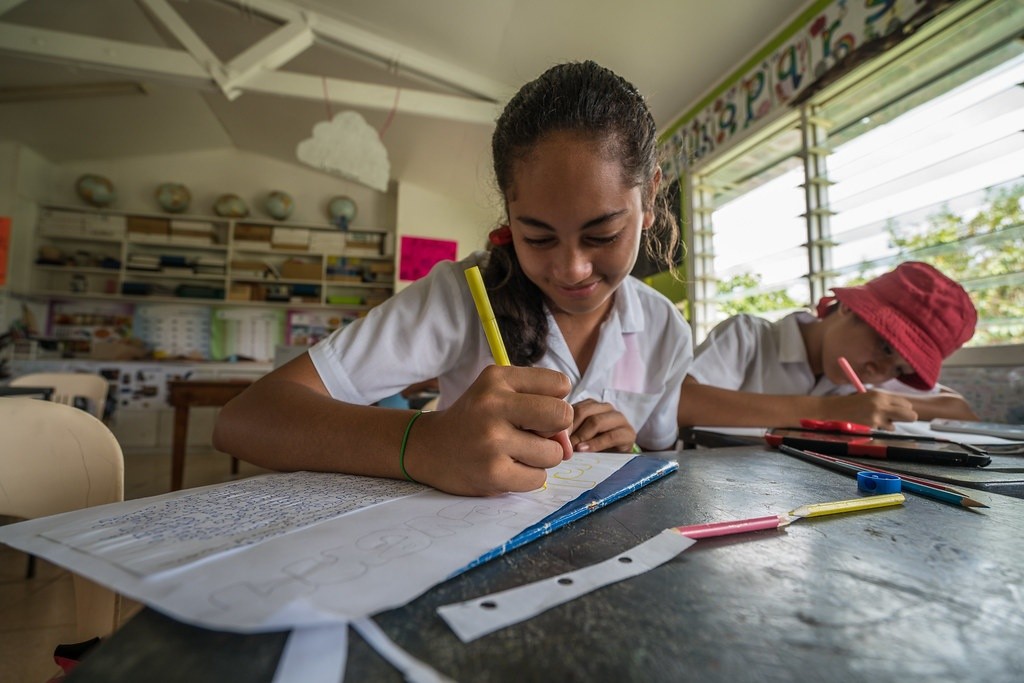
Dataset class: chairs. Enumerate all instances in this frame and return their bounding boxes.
[0,373,127,644]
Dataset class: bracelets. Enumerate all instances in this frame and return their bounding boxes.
[400,412,422,485]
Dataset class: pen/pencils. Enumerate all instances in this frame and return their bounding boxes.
[671,514,790,538]
[780,446,862,480]
[835,461,991,508]
[804,450,970,498]
[838,357,867,394]
[464,265,548,490]
[788,492,906,517]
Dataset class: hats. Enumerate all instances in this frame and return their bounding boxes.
[829,261,977,392]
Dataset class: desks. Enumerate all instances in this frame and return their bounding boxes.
[166,377,258,493]
[80,447,1024,683]
[693,425,1024,500]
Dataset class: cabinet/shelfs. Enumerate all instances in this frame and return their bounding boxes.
[3,200,394,411]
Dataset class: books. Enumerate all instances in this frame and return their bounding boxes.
[127,254,225,276]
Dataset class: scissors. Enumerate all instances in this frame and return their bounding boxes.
[800,418,918,436]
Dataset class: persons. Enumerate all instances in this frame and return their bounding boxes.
[677,261,982,431]
[212,60,694,497]
